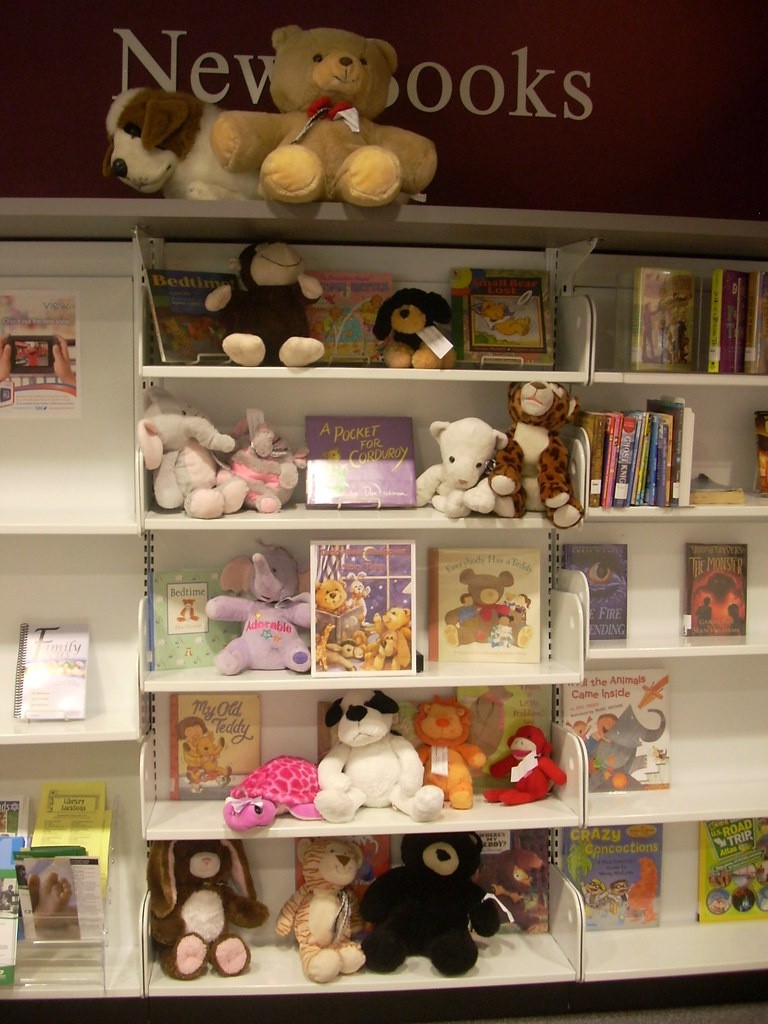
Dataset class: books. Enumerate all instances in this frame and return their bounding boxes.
[630,266,767,375]
[564,669,669,794]
[165,684,559,802]
[578,395,744,510]
[562,543,628,642]
[295,829,549,935]
[306,416,414,511]
[561,824,663,930]
[682,544,747,638]
[696,818,768,923]
[16,623,91,721]
[144,266,558,372]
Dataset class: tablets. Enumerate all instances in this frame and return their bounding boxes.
[7,334,56,374]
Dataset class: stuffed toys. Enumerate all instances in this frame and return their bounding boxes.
[223,689,487,831]
[358,831,500,977]
[484,726,567,806]
[205,544,311,675]
[276,838,366,983]
[147,839,269,981]
[103,25,438,207]
[205,241,326,368]
[138,387,307,519]
[373,287,456,369]
[416,381,584,530]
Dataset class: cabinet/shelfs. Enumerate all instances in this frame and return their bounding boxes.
[0,197,768,1023]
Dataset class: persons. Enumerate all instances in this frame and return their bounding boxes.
[28,872,76,929]
[0,333,77,386]
